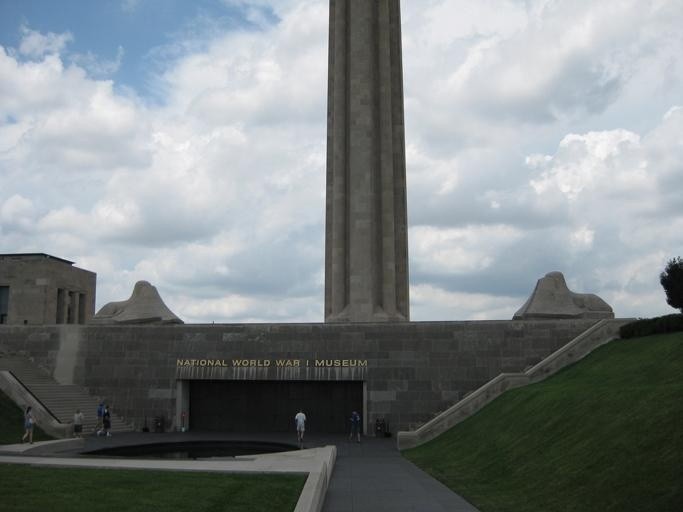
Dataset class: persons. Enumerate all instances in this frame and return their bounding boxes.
[294,406,307,441]
[19,404,35,443]
[96,402,104,436]
[95,402,112,437]
[66,407,83,437]
[346,408,361,443]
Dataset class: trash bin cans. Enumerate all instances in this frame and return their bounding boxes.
[376,418,386,441]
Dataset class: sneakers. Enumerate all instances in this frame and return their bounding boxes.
[91,428,113,438]
